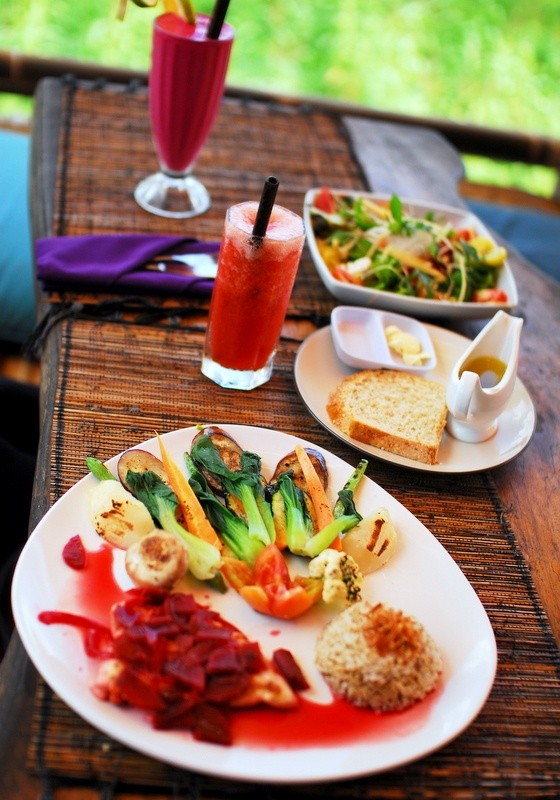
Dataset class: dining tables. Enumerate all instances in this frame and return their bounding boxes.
[1,77,560,800]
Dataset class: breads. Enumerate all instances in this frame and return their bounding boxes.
[321,370,455,468]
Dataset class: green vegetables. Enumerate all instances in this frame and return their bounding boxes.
[113,430,371,581]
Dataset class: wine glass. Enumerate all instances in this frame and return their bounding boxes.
[134,8,234,222]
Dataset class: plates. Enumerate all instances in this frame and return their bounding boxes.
[303,184,517,322]
[329,303,436,373]
[11,421,498,789]
[295,319,535,475]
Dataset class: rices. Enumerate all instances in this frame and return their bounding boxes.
[314,602,443,716]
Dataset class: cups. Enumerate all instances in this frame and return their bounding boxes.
[443,308,525,442]
[200,198,309,392]
